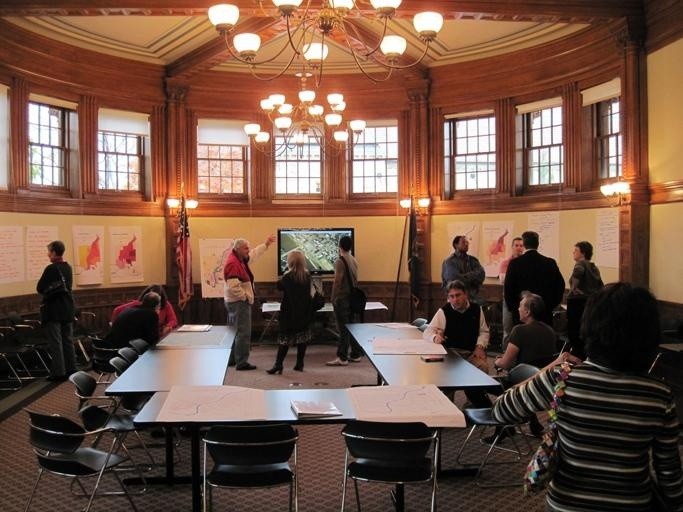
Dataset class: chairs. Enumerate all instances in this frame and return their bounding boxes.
[117,346,139,365]
[455,363,554,488]
[69,370,156,474]
[341,420,441,512]
[129,338,149,356]
[202,420,299,512]
[0,311,99,392]
[24,406,148,512]
[108,356,129,378]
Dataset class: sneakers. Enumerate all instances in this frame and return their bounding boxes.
[46,375,56,382]
[483,426,517,443]
[236,363,256,371]
[347,355,361,361]
[326,358,349,365]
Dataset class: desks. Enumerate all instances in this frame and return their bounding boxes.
[133,383,466,512]
[257,301,388,346]
[103,323,239,483]
[344,318,503,480]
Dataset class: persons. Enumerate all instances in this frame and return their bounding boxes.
[325,236,361,367]
[265,251,316,375]
[223,233,277,371]
[110,291,161,349]
[442,234,485,302]
[565,241,604,363]
[421,280,490,409]
[112,284,177,337]
[490,281,683,512]
[499,237,524,352]
[36,240,76,381]
[464,294,556,445]
[503,230,565,328]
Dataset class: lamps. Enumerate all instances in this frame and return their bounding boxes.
[165,183,199,210]
[206,0,444,87]
[399,191,432,214]
[600,175,630,208]
[243,21,367,158]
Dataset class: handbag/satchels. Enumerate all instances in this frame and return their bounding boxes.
[522,361,573,496]
[54,264,76,322]
[309,276,326,310]
[338,255,366,316]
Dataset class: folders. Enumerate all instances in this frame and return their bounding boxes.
[290,398,342,420]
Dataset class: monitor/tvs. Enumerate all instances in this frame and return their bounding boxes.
[277,227,356,276]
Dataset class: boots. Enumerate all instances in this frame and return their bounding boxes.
[267,360,283,375]
[294,361,304,372]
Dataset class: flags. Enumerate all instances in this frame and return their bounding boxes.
[175,186,195,311]
[407,212,424,311]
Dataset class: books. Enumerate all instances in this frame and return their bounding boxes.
[372,337,448,356]
[177,324,213,332]
[290,400,343,418]
